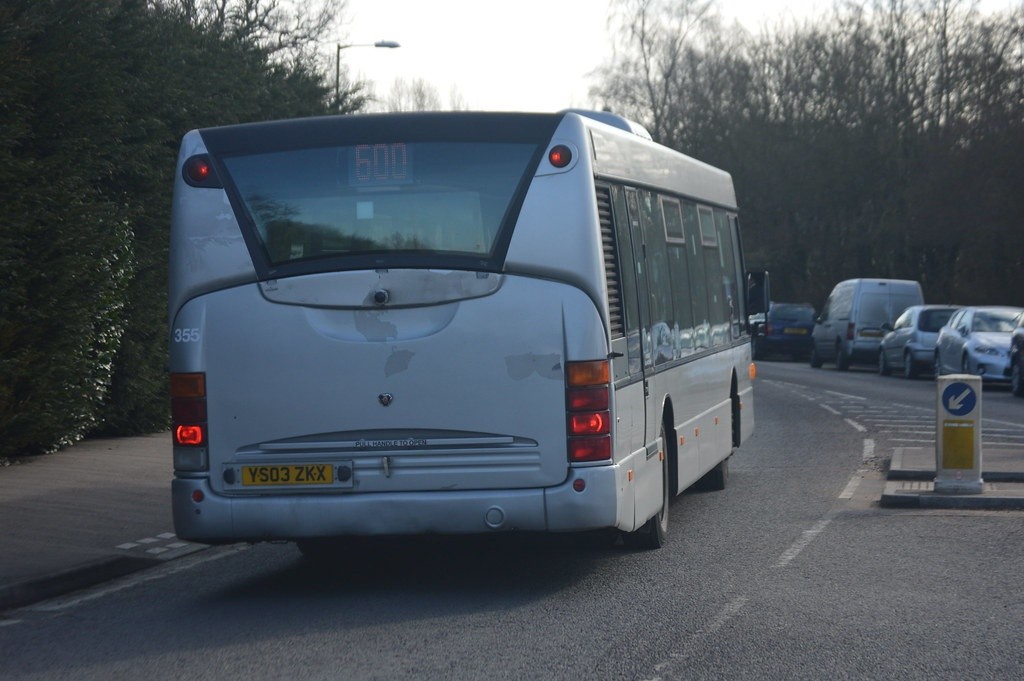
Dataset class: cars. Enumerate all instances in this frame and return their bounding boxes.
[1002,310,1024,396]
[749,301,819,360]
[932,306,1024,384]
[877,304,967,379]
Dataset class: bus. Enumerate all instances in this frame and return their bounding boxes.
[168,107,770,550]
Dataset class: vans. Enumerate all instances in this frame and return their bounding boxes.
[810,278,925,371]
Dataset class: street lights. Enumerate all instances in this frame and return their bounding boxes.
[332,39,403,112]
